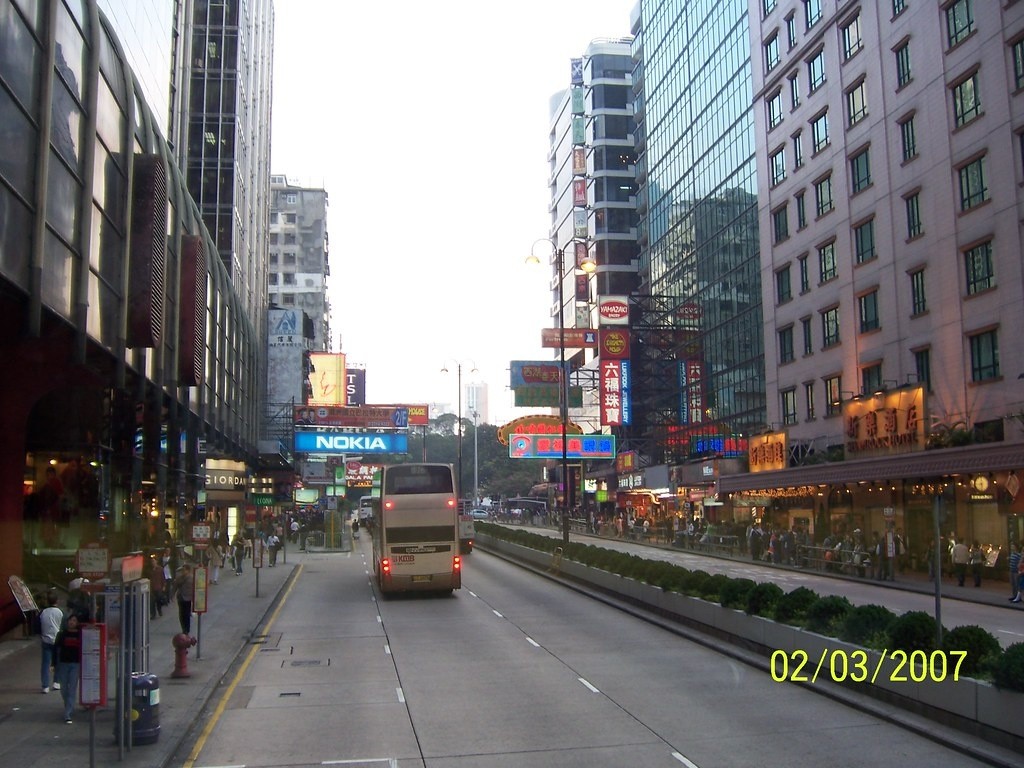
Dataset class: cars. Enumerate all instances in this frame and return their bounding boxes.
[467,508,489,519]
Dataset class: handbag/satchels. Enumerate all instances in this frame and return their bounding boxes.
[982,553,986,562]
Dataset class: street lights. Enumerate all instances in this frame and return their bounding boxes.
[422,401,438,462]
[440,361,480,498]
[525,236,600,543]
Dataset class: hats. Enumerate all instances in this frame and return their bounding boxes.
[854,528,860,532]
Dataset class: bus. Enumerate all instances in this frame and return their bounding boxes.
[459,499,475,553]
[368,462,462,598]
[357,495,371,527]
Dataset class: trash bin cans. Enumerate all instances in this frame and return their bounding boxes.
[114,671,162,746]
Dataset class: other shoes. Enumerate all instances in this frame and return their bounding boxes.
[214,579,218,585]
[41,687,49,693]
[64,714,72,724]
[158,610,162,616]
[209,579,212,584]
[300,548,305,550]
[167,600,170,603]
[233,568,234,570]
[269,564,272,566]
[1012,600,1018,604]
[959,583,963,585]
[150,613,154,619]
[1009,596,1015,601]
[53,682,60,689]
[974,584,981,587]
[171,598,174,602]
[236,572,241,575]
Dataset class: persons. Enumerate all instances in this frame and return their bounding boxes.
[491,504,1024,605]
[294,408,318,431]
[41,509,359,725]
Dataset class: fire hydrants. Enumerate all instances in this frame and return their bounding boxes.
[172,633,198,679]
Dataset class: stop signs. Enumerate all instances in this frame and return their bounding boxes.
[244,505,257,524]
